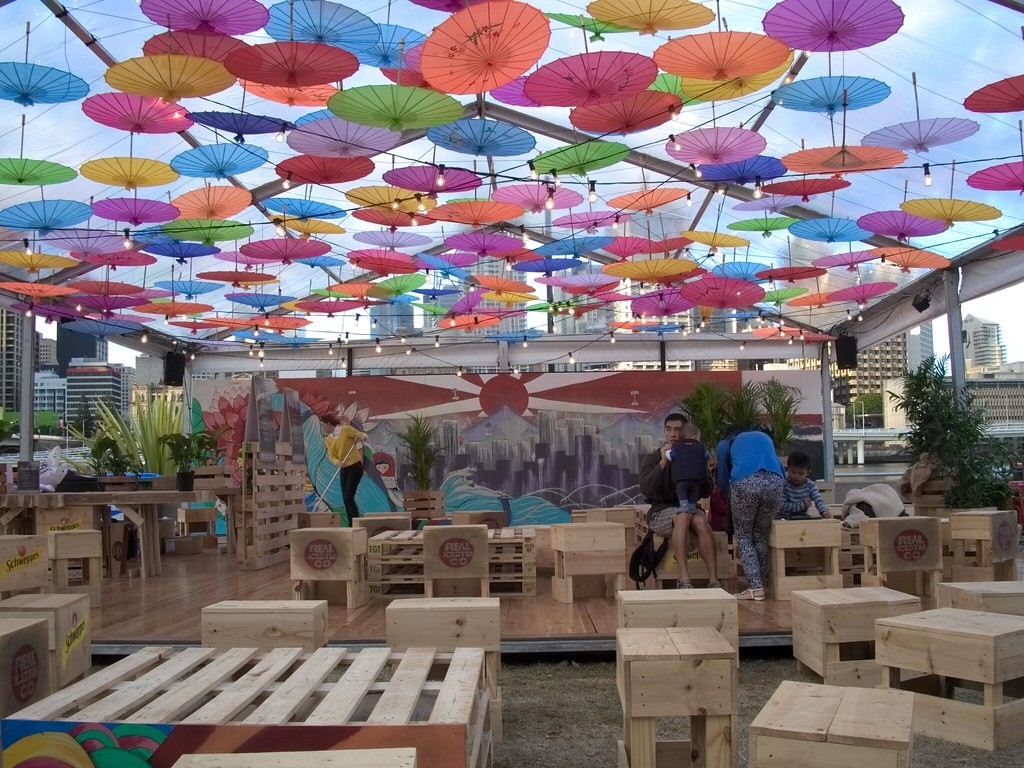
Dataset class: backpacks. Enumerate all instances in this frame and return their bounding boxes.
[707,481,735,544]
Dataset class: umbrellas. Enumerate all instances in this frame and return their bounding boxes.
[0,0,1024,347]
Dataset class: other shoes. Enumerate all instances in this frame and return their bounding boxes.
[677,578,695,590]
[709,581,726,591]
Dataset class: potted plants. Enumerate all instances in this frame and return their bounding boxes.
[155,424,265,500]
[397,411,447,513]
[96,447,145,492]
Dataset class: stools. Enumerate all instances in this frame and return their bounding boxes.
[0,504,1023,768]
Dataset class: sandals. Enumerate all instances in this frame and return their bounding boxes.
[732,587,765,601]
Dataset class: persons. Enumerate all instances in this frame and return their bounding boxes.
[321,415,368,527]
[774,452,830,519]
[640,413,724,589]
[707,424,786,600]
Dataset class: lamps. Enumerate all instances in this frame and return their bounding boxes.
[912,294,931,316]
